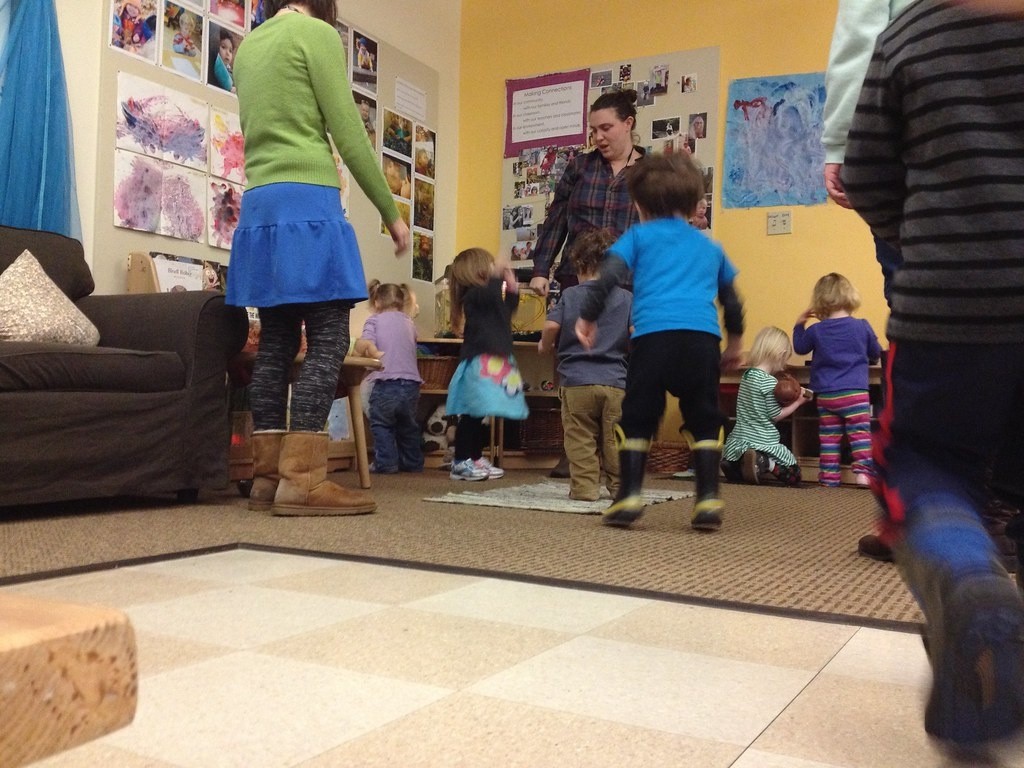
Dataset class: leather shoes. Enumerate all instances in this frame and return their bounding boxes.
[551,456,570,478]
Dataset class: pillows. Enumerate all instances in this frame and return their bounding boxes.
[0,249,100,348]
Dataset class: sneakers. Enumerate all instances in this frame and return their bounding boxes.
[452,458,504,480]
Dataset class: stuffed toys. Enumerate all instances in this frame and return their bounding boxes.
[424,402,461,451]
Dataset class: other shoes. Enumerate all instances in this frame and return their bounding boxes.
[858,535,895,561]
[720,449,769,484]
[369,462,398,474]
[856,473,875,489]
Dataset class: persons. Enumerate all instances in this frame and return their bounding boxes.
[537,227,633,501]
[822,0,1024,740]
[575,153,744,531]
[353,35,435,282]
[112,0,266,94]
[444,248,528,482]
[359,279,425,474]
[794,274,882,488]
[504,144,576,314]
[224,0,412,518]
[593,65,710,229]
[529,88,654,478]
[721,326,813,485]
[349,339,386,359]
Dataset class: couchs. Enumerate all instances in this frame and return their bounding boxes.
[0,225,249,512]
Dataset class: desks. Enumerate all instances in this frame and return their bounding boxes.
[226,352,382,488]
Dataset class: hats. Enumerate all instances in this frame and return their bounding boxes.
[119,0,142,24]
[359,38,367,47]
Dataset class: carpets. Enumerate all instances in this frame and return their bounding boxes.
[0,451,926,635]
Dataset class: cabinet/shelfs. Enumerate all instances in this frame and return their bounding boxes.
[416,339,554,468]
[718,364,884,484]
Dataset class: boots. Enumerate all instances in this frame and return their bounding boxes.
[601,424,653,523]
[679,423,726,525]
[249,430,379,515]
[887,494,1024,744]
